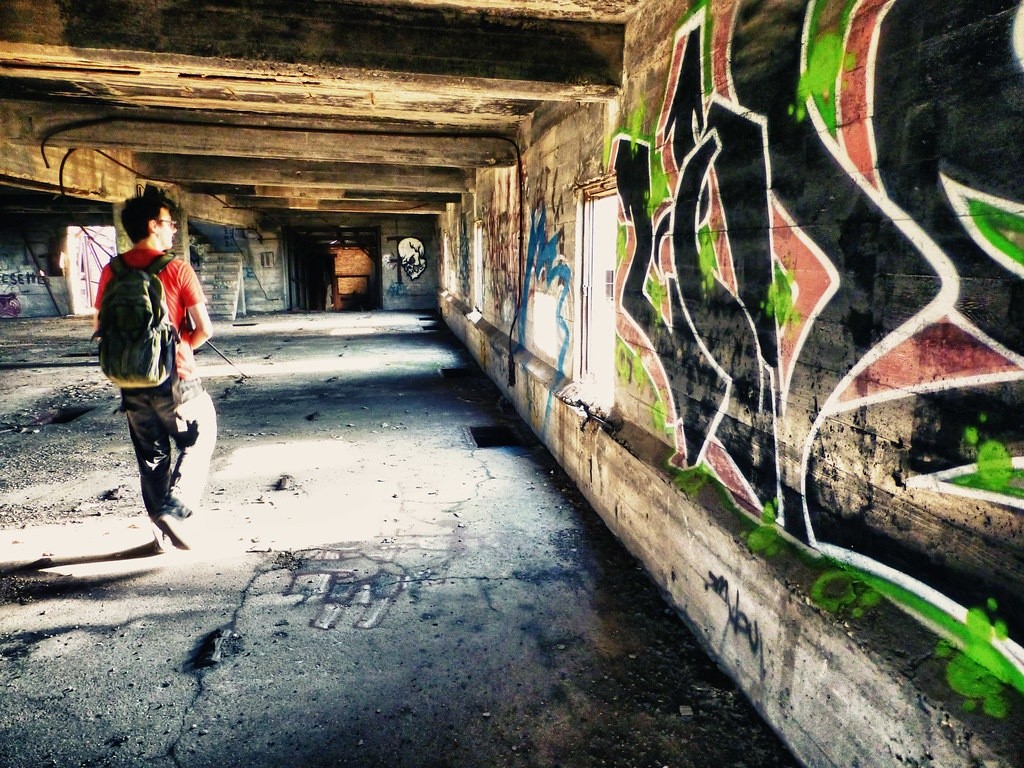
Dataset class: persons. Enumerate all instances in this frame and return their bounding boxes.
[92,195,218,553]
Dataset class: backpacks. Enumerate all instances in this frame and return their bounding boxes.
[89,250,176,390]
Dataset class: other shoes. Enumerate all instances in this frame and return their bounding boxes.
[151,520,172,552]
[156,514,193,551]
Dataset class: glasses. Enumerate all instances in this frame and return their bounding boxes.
[161,220,177,227]
